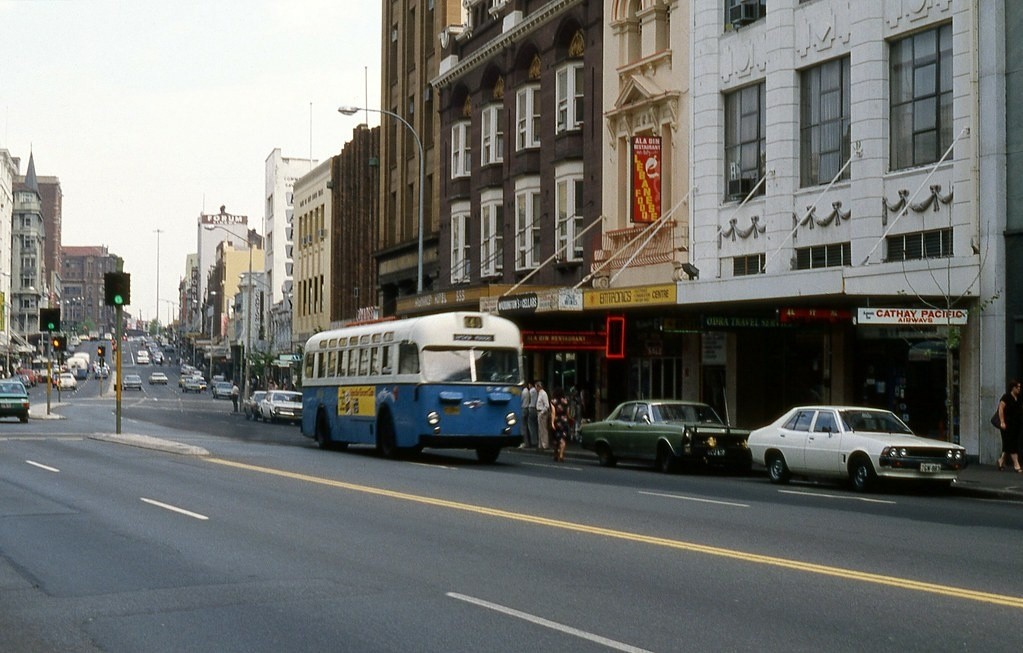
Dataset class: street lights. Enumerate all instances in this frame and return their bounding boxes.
[204,225,261,399]
[338,104,424,295]
[153,229,164,335]
[193,273,271,391]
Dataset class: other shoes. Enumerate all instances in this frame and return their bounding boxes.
[1013,467,1023,473]
[995,460,1004,472]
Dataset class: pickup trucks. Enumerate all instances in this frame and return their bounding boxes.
[243,390,303,424]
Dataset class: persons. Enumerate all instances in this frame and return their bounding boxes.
[231,382,240,413]
[518,376,588,462]
[849,413,863,432]
[35,371,39,387]
[997,379,1023,473]
[266,379,297,391]
[159,356,180,366]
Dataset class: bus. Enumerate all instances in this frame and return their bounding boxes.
[300,311,525,464]
[32,360,59,382]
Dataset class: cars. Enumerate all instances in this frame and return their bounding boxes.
[93,361,110,379]
[148,372,168,386]
[128,335,174,365]
[746,405,966,493]
[0,379,30,423]
[8,365,77,391]
[579,399,755,475]
[122,375,142,391]
[63,332,113,359]
[178,364,234,398]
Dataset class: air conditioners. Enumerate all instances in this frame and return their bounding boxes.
[429,0,434,11]
[392,29,397,42]
[425,87,432,103]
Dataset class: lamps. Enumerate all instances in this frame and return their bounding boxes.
[369,156,381,166]
[327,180,334,189]
[682,263,699,281]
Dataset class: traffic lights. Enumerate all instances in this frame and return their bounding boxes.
[98,347,103,356]
[40,308,60,332]
[104,272,130,306]
[53,337,66,351]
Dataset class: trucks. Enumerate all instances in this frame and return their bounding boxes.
[66,352,90,380]
[136,350,150,364]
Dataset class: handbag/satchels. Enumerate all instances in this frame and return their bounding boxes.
[991,407,1000,429]
